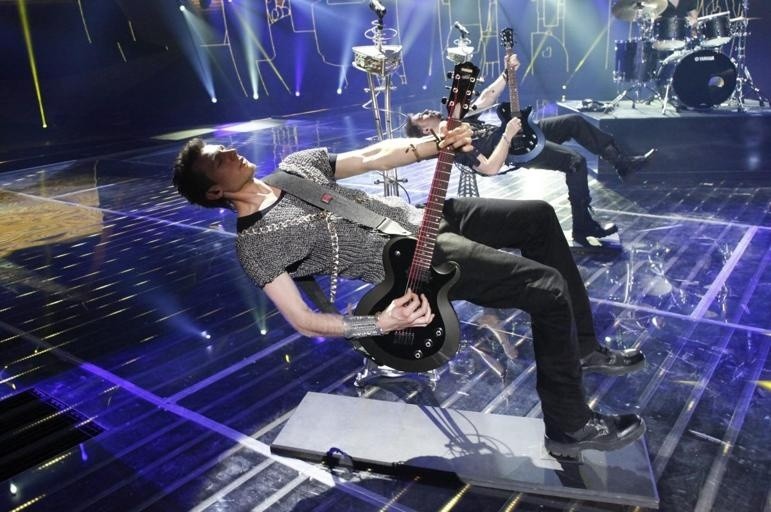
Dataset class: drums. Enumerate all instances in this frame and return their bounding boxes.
[650,16,687,50]
[697,12,732,48]
[658,51,738,111]
[613,37,658,84]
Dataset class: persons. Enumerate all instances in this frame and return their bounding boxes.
[172,120,648,457]
[656,0,698,99]
[405,54,660,248]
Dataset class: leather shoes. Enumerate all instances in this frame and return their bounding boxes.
[580,344,646,375]
[573,223,617,240]
[544,412,646,458]
[616,149,658,179]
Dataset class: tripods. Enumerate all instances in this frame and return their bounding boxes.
[608,86,672,113]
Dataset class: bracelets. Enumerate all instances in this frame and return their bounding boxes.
[501,68,509,81]
[343,311,389,340]
[430,127,455,156]
[405,143,422,162]
[502,133,511,148]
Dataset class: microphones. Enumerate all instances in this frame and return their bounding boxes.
[455,21,469,35]
[371,1,387,14]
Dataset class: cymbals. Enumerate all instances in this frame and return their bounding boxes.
[728,16,763,22]
[613,0,668,21]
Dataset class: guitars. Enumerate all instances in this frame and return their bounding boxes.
[492,29,545,167]
[355,61,480,373]
